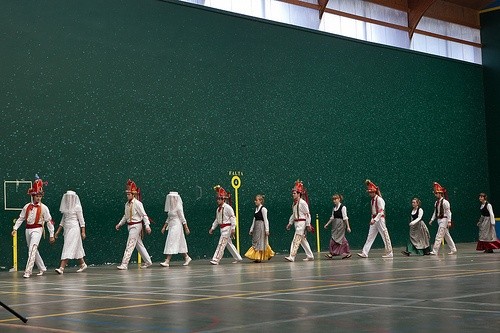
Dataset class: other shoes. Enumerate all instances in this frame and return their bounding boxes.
[324,254,332,258]
[357,252,368,258]
[37,270,44,276]
[117,265,128,270]
[253,260,262,263]
[141,262,152,268]
[401,251,409,256]
[76,264,88,272]
[232,259,243,264]
[160,262,170,267]
[430,251,438,255]
[24,273,30,277]
[285,257,294,262]
[343,254,352,259]
[303,257,314,261]
[382,255,393,258]
[56,269,63,274]
[483,250,494,253]
[209,261,219,265]
[183,257,192,266]
[448,251,457,255]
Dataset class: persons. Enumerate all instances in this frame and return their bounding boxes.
[12,179,54,278]
[244,194,275,263]
[285,181,314,262]
[323,194,352,258]
[115,180,152,270]
[476,193,500,253]
[54,190,87,275]
[357,179,393,258]
[428,182,457,255]
[159,192,192,267]
[401,197,431,256]
[208,185,243,265]
